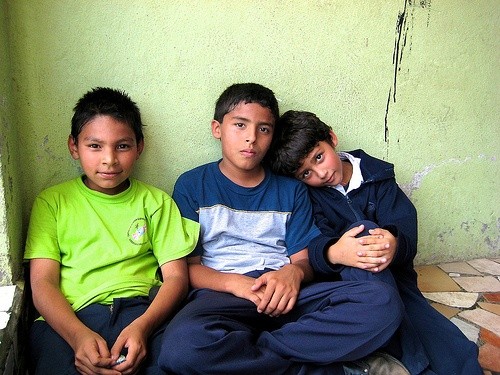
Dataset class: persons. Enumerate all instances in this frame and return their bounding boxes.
[23,87,201,375]
[155,83,410,375]
[261,110,484,375]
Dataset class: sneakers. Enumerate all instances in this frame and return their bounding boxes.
[342,350,410,375]
